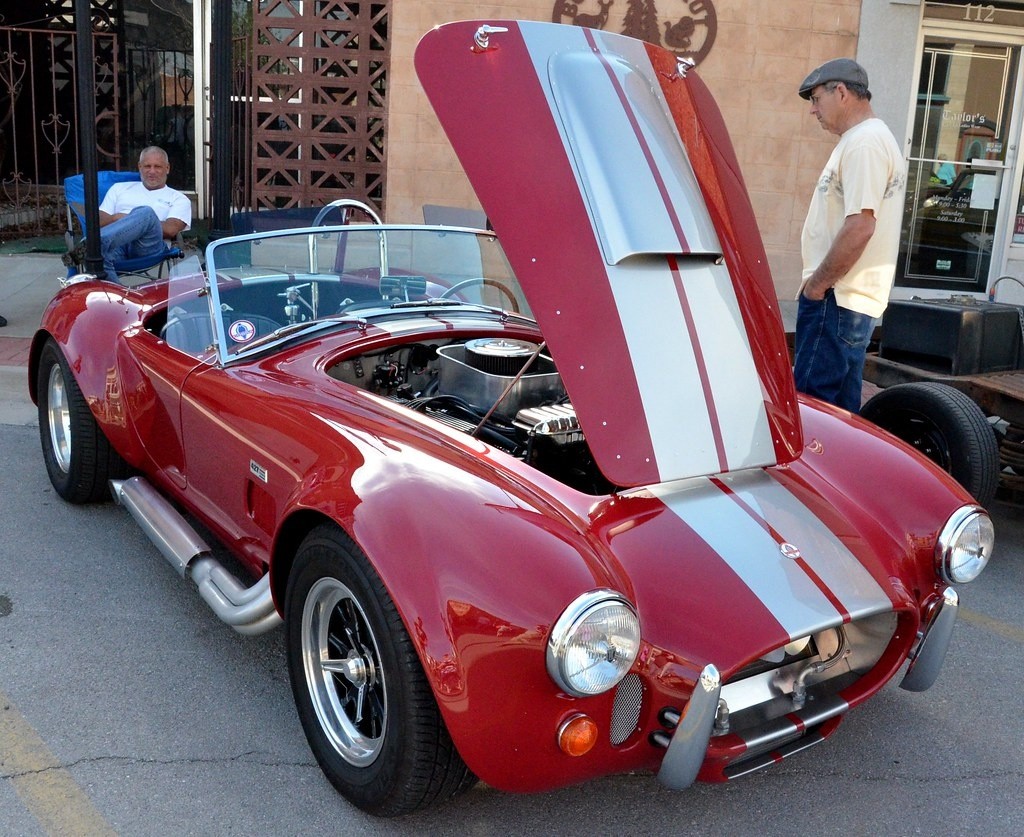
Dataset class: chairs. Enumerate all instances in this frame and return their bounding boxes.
[160,312,285,354]
[64,171,186,285]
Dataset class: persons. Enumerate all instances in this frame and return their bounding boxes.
[794,58,905,412]
[61,146,192,285]
[936,154,956,186]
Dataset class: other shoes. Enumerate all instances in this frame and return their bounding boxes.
[60,240,88,268]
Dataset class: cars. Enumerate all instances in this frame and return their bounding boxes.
[24,17,996,826]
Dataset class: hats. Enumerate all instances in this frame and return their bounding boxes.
[798,58,869,101]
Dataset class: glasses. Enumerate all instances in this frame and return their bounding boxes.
[809,85,838,106]
